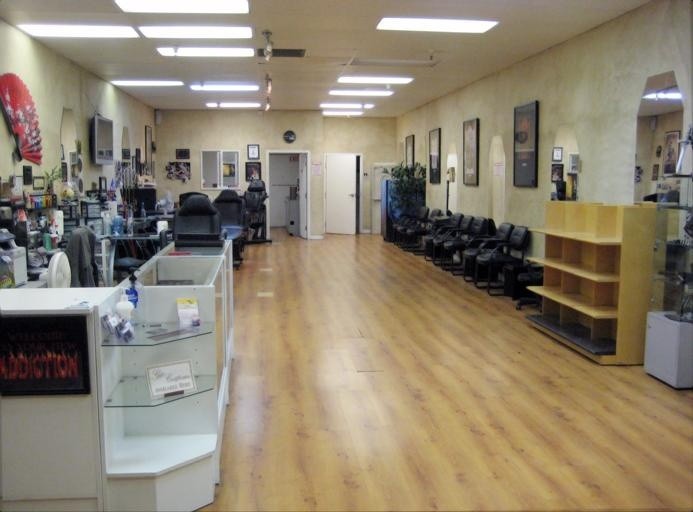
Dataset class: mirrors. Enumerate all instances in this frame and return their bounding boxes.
[633,71,684,206]
[60,107,79,182]
[122,127,130,183]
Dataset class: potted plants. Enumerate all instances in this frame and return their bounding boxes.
[44,165,59,194]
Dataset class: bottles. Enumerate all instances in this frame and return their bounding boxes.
[101,199,148,238]
[11,179,58,251]
[555,172,577,201]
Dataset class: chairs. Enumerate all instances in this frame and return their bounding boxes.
[392,202,544,311]
[46,179,272,287]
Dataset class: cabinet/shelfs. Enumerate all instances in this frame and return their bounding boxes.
[524,174,693,389]
[93,239,235,512]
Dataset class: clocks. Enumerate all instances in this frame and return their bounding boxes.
[283,130,296,143]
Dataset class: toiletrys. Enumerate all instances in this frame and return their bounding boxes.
[116,286,137,333]
[120,268,148,325]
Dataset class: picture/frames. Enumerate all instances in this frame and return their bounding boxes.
[132,147,144,176]
[165,125,261,182]
[33,176,45,190]
[406,100,580,188]
[61,144,78,182]
[652,129,681,180]
[122,149,130,159]
